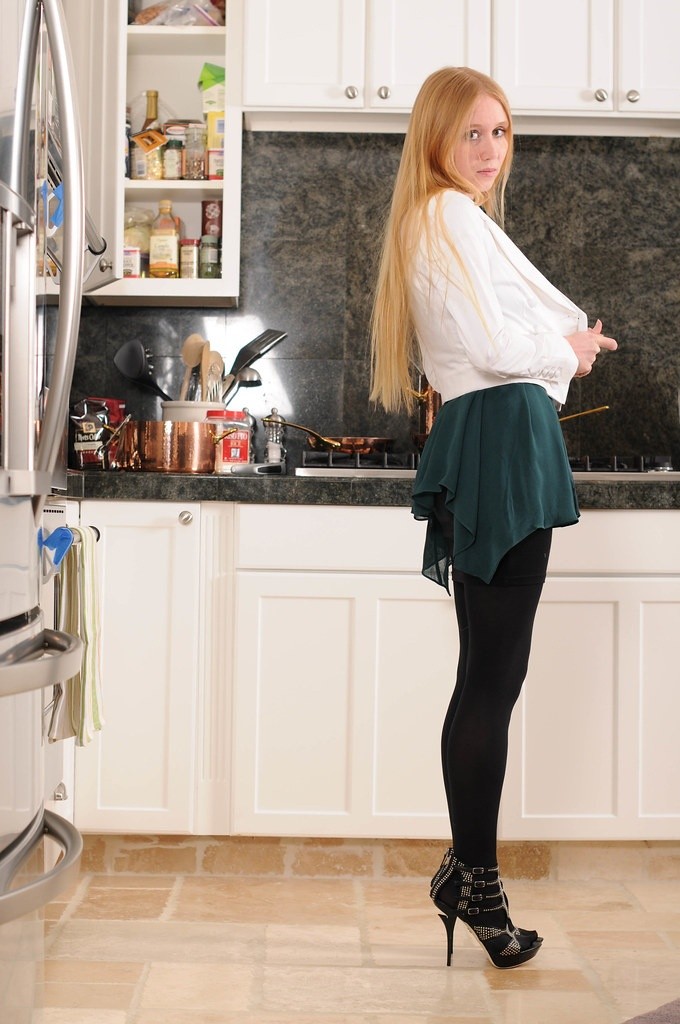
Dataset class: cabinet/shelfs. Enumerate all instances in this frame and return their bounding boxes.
[63,500,680,841]
[243,0,477,133]
[85,0,244,308]
[477,0,680,138]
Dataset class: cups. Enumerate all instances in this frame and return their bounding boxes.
[160,400,226,421]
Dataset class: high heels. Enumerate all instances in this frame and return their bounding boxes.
[429,848,544,969]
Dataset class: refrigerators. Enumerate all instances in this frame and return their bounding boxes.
[0,0,86,1024]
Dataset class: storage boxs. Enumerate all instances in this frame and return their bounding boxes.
[198,63,224,179]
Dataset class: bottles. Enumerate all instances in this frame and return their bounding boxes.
[202,410,249,475]
[125,91,224,180]
[199,235,218,278]
[180,238,200,278]
[149,200,179,278]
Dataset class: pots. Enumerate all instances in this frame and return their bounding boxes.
[261,418,396,454]
[103,421,238,473]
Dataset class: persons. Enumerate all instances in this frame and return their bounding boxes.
[369,68,617,968]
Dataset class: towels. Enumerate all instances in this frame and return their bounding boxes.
[48,525,101,746]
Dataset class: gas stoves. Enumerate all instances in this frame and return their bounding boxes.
[295,441,680,481]
